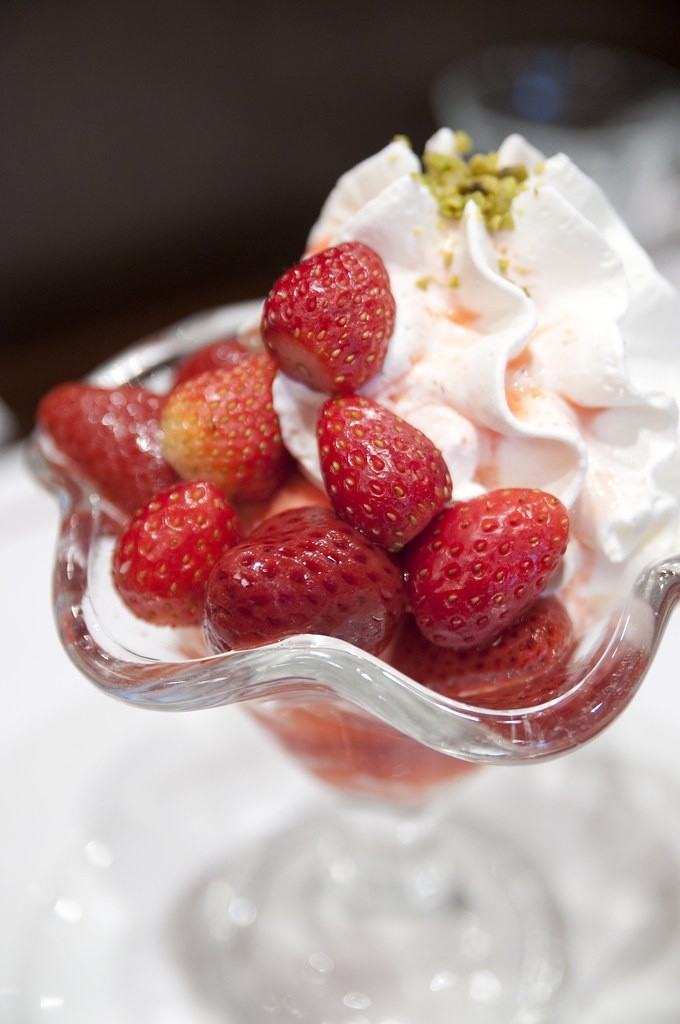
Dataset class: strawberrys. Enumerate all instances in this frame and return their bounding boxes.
[36,241,576,722]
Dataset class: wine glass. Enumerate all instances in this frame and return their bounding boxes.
[21,287,680,1024]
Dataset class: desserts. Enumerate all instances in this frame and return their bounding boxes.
[244,121,680,684]
